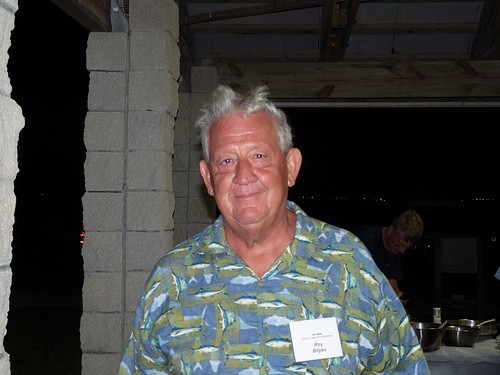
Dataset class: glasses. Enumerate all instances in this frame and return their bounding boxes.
[394,244,413,249]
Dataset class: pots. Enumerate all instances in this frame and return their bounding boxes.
[443,318,496,347]
[411,322,443,352]
